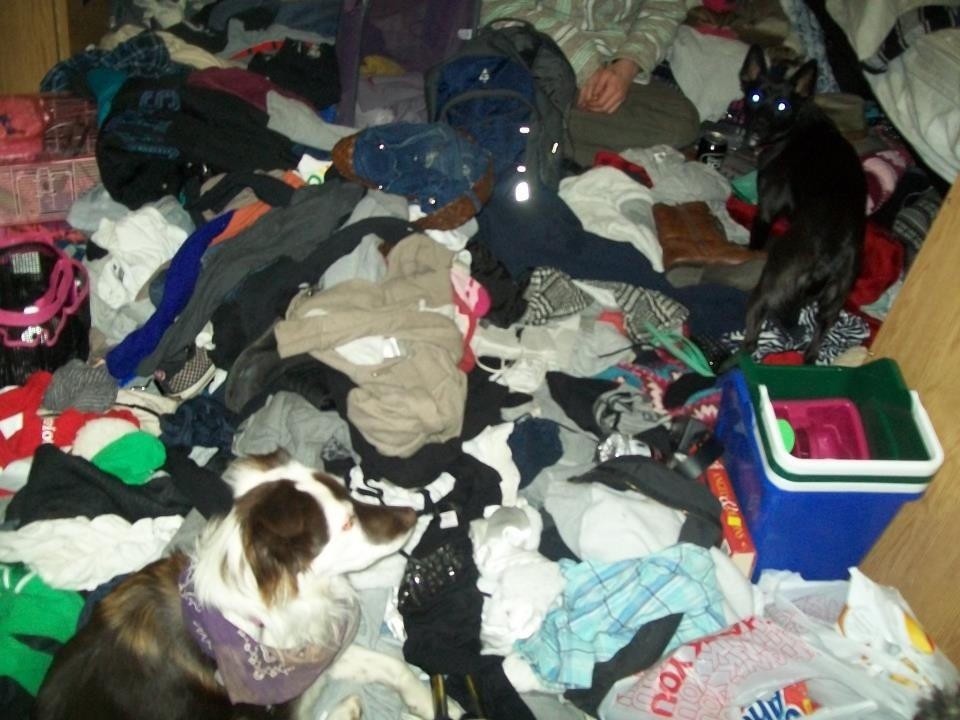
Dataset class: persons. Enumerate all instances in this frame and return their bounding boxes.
[479,0,708,165]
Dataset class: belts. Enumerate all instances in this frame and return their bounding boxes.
[230,39,314,62]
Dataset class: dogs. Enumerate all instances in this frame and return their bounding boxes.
[737,45,868,366]
[31,445,417,720]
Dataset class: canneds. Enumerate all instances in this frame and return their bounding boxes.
[695,131,728,171]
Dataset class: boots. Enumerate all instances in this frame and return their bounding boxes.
[653,202,763,293]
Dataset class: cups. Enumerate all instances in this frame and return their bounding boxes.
[816,92,863,145]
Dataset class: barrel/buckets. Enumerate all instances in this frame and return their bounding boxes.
[714,356,944,585]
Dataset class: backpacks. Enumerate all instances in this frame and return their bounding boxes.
[423,18,579,196]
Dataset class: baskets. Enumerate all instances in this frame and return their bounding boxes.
[332,124,492,231]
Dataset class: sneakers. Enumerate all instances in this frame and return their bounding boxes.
[154,346,216,402]
